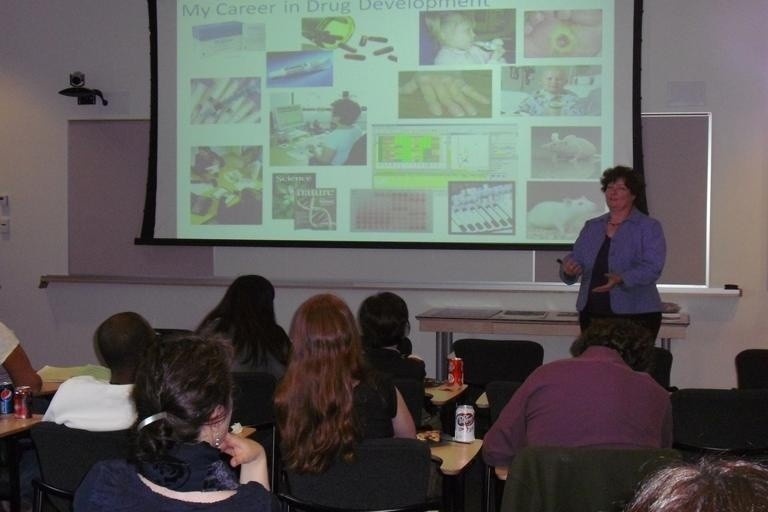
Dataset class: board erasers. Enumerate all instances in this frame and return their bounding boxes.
[725,284,738,290]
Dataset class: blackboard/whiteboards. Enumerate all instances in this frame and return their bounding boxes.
[210,247,535,286]
[533,112,712,287]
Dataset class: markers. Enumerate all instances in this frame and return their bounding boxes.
[556,258,563,265]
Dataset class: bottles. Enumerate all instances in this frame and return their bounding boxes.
[453,405,476,444]
[0,381,13,415]
[447,357,464,386]
[14,386,31,419]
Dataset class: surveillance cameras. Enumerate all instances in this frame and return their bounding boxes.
[70,72,85,87]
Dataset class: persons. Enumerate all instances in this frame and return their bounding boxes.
[426,11,506,66]
[43,311,152,432]
[0,321,42,400]
[399,71,490,117]
[356,292,434,436]
[191,147,225,177]
[483,317,674,479]
[73,336,288,512]
[310,99,361,166]
[237,148,260,180]
[274,294,417,472]
[195,275,288,381]
[506,66,592,116]
[560,165,666,349]
[523,9,602,59]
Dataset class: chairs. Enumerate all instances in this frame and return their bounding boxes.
[230,372,280,444]
[650,346,677,390]
[451,339,546,439]
[725,351,768,388]
[385,379,428,432]
[501,443,683,511]
[29,422,114,512]
[277,435,442,511]
[670,389,768,462]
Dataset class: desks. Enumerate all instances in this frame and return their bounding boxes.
[424,382,467,407]
[37,365,110,396]
[429,433,485,475]
[0,413,47,438]
[415,306,696,381]
